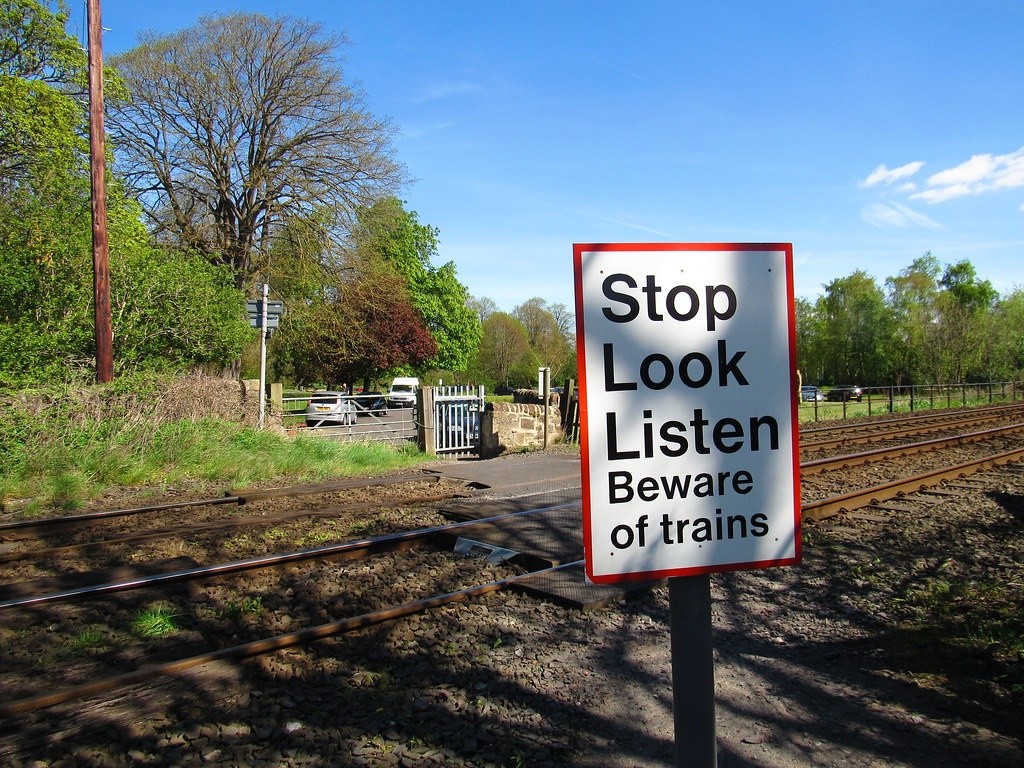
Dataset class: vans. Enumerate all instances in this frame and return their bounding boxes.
[388,377,422,407]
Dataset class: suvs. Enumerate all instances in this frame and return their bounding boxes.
[827,383,862,403]
[494,385,514,396]
[304,392,359,427]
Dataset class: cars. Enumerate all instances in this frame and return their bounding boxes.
[552,384,579,397]
[439,403,488,451]
[800,385,824,401]
[355,391,388,417]
